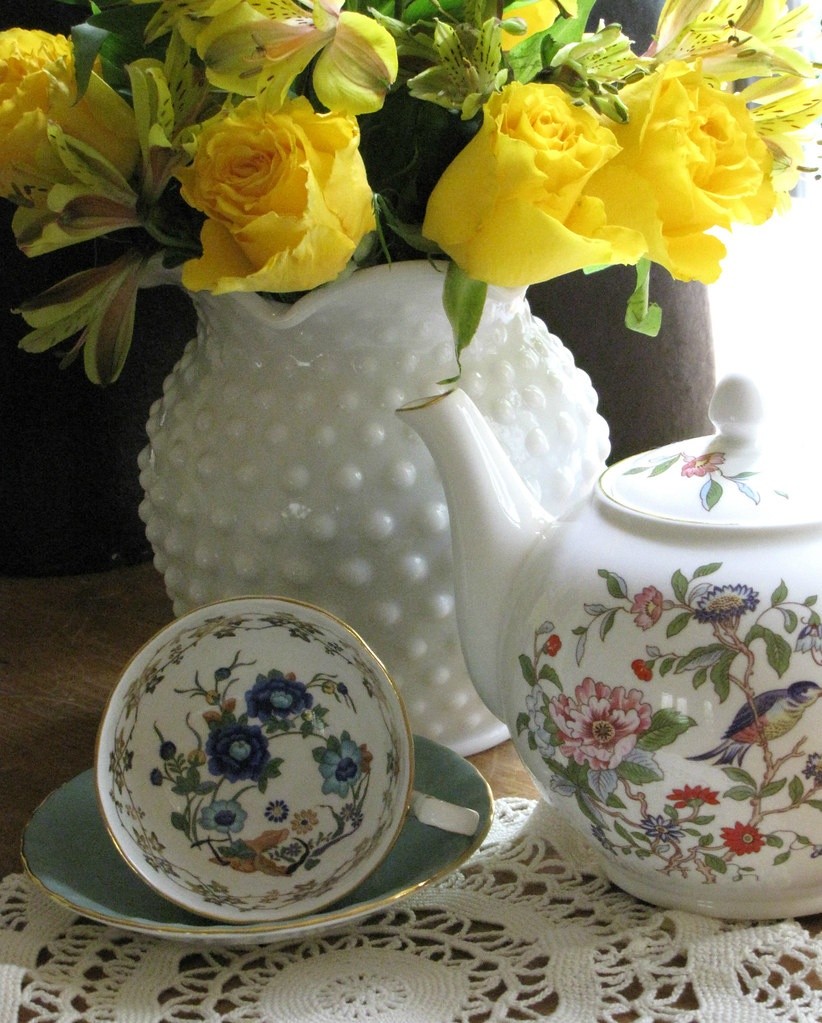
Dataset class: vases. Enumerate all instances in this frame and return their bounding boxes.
[138,254,610,757]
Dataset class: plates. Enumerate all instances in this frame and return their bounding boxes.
[21,734,494,944]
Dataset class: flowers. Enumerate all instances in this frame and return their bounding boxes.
[0,0,822,387]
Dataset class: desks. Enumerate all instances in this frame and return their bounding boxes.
[1,560,822,1023]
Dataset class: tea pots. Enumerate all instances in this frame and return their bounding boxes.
[396,375,822,919]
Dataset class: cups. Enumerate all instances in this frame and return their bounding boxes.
[97,597,479,922]
